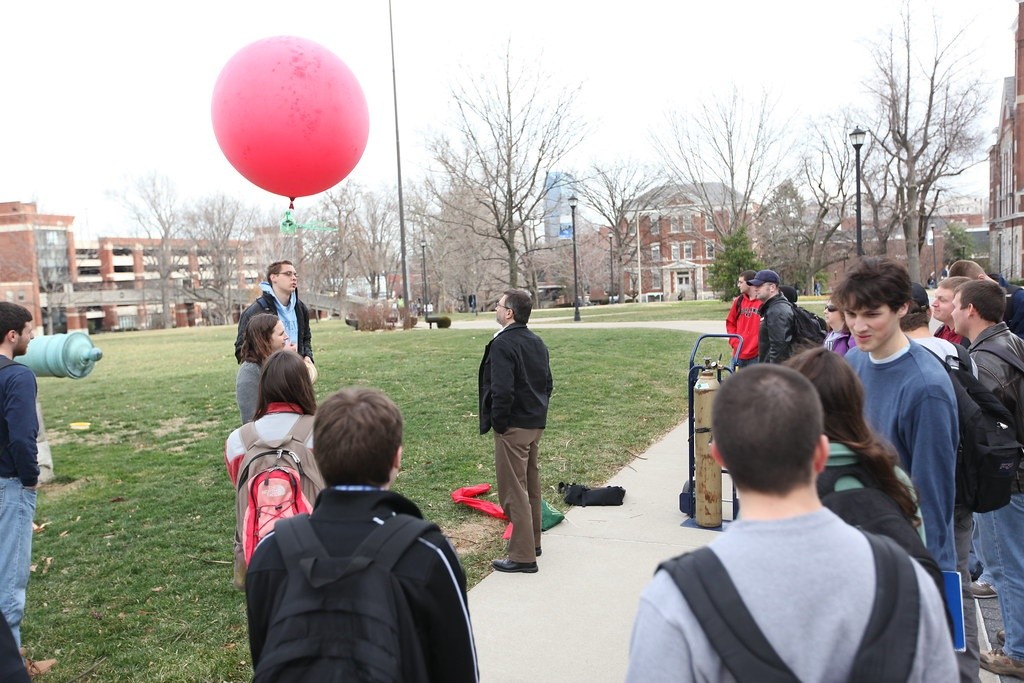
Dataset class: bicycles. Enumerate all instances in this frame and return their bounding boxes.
[397,305,405,320]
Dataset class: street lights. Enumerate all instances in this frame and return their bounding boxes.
[833,268,838,280]
[420,239,427,318]
[567,193,581,321]
[930,222,938,289]
[849,125,866,258]
[608,231,614,304]
[419,253,424,317]
[962,243,965,260]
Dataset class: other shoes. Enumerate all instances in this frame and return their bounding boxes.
[25,659,57,676]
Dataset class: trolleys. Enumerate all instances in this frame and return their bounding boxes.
[679,335,744,523]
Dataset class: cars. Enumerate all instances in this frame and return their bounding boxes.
[111,326,140,332]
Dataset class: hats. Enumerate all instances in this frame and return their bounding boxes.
[746,270,779,286]
[909,282,929,312]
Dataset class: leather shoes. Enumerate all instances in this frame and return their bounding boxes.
[491,559,538,573]
[535,547,542,556]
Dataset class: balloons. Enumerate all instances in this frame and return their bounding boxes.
[210,35,368,211]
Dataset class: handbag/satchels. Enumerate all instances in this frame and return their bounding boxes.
[557,481,625,507]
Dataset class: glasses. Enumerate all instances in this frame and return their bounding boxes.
[496,301,514,315]
[824,305,839,312]
[273,271,299,277]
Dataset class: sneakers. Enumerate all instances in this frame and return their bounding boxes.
[971,580,997,598]
[980,648,1024,680]
[997,630,1005,646]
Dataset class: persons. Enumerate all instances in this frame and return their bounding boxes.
[223,314,480,683]
[0,302,58,677]
[625,257,1024,683]
[234,260,315,368]
[477,286,553,573]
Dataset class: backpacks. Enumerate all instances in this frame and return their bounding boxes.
[920,340,1024,513]
[250,512,441,683]
[235,413,326,592]
[815,457,958,649]
[766,300,828,343]
